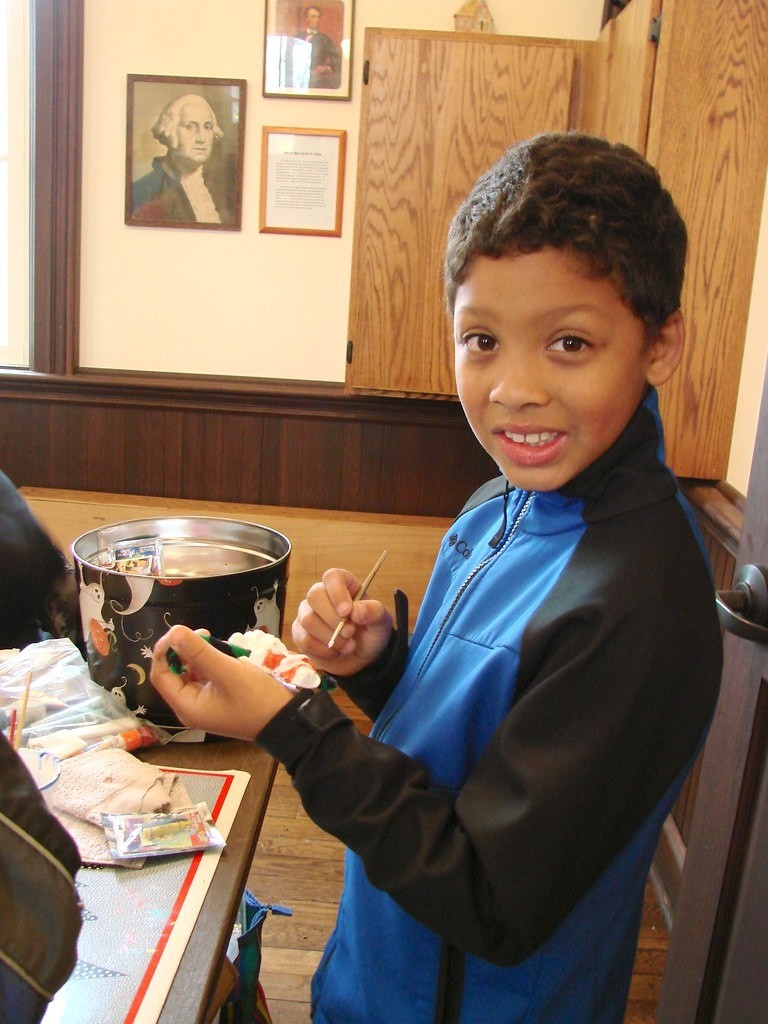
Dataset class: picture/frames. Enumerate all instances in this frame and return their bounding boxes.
[257,124,348,234]
[263,1,351,94]
[123,75,245,233]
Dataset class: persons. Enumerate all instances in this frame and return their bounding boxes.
[133,92,235,225]
[290,6,342,88]
[148,136,726,1024]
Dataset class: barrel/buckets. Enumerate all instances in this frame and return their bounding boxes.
[73,516,290,744]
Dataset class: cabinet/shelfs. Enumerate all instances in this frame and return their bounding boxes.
[346,0,767,460]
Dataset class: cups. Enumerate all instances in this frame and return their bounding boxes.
[17,748,61,813]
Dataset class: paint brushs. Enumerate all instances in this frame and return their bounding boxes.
[327,549,390,649]
[14,670,35,752]
[9,709,17,745]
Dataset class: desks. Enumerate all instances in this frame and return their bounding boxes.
[42,715,276,1024]
